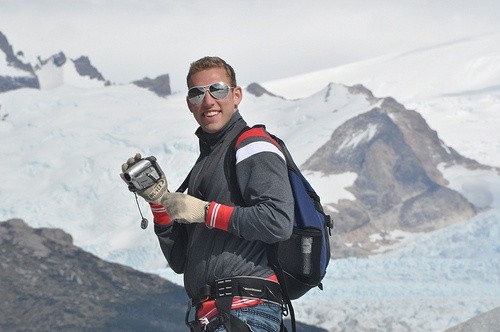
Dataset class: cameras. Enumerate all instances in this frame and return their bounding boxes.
[120,156,162,192]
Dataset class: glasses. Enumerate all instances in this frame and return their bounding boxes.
[188,82,234,105]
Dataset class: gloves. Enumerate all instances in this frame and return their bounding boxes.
[160,192,209,224]
[119,153,168,203]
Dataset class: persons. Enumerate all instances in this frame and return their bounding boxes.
[119,57,293,332]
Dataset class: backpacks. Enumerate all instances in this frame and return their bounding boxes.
[224,124,334,301]
